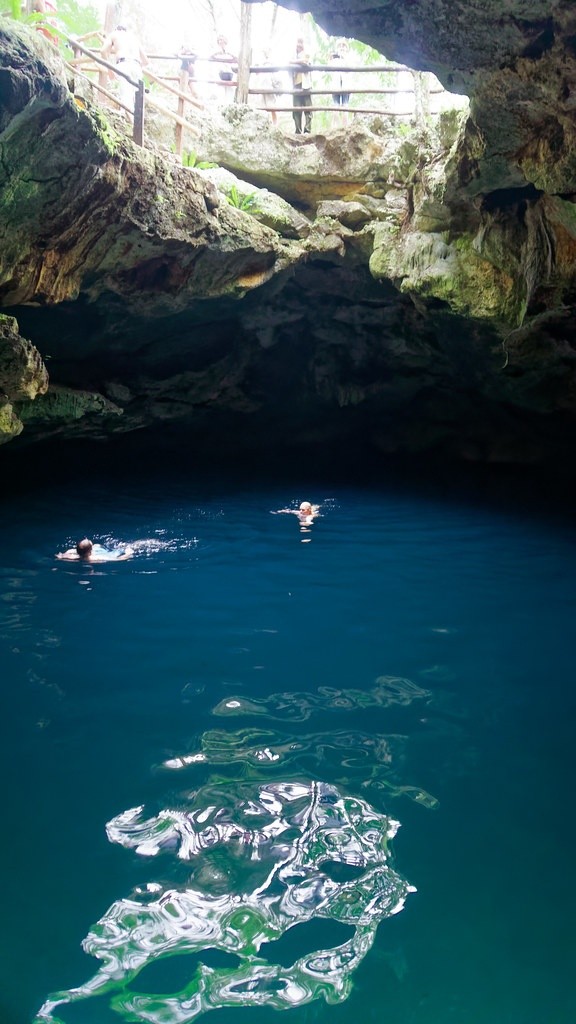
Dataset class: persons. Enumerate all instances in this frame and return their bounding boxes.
[326,37,353,126]
[277,501,311,515]
[35,0,315,134]
[59,538,135,562]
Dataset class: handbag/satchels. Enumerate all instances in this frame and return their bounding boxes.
[271,77,284,96]
[219,72,233,81]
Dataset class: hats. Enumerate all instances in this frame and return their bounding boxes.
[335,38,349,53]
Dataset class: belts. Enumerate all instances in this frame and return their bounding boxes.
[116,58,141,65]
